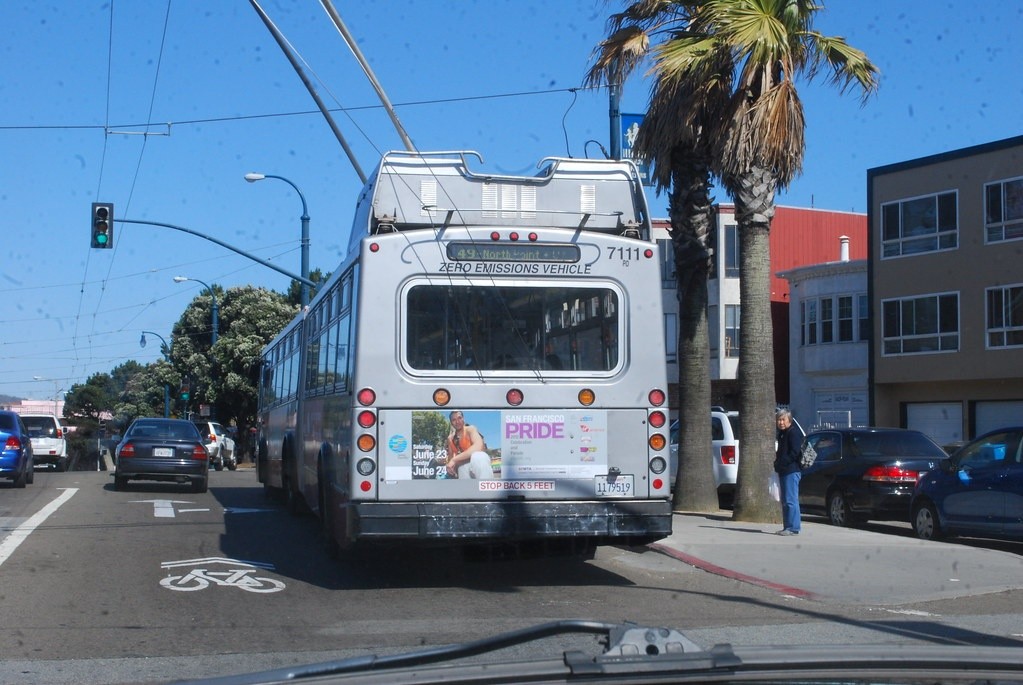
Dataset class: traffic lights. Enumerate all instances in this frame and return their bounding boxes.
[180,377,191,402]
[92,200,115,252]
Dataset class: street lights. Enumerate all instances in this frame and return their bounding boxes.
[34,377,58,416]
[140,331,171,417]
[173,277,219,347]
[244,173,310,306]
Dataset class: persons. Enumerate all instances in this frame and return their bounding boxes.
[224,416,258,465]
[411,411,495,480]
[774,408,806,535]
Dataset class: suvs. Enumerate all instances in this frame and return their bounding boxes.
[18,414,69,472]
[668,405,807,508]
[193,419,237,471]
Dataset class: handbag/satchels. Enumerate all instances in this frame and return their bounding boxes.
[798,440,817,469]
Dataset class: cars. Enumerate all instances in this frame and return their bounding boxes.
[111,416,210,493]
[799,426,951,527]
[0,409,36,489]
[907,426,1023,542]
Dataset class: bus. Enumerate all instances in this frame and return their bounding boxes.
[247,149,674,561]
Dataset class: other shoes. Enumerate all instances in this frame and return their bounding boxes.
[776,530,783,534]
[780,529,799,535]
